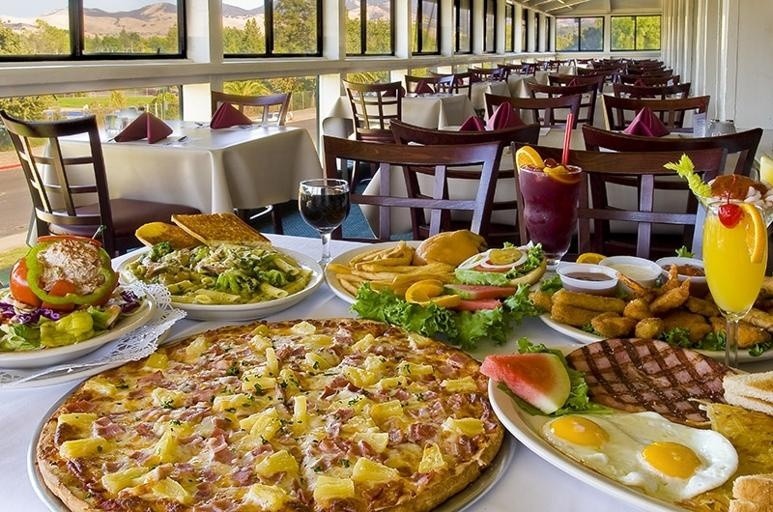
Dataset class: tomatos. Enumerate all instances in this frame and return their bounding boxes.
[9,234,121,311]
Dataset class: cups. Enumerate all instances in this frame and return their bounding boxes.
[103,114,131,141]
[517,161,583,273]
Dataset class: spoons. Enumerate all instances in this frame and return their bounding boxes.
[162,135,189,146]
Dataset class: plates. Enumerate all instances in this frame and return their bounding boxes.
[0,296,153,373]
[487,361,697,510]
[324,238,540,333]
[114,245,325,324]
[536,307,771,363]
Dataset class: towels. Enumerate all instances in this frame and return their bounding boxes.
[213,95,255,133]
[109,111,173,144]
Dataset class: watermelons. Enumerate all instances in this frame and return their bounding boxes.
[478,351,571,415]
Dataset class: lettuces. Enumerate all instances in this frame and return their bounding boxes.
[495,336,589,417]
[349,283,535,351]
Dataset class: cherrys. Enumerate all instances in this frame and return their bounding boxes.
[718,174,743,227]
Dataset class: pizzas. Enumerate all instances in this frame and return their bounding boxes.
[29,315,503,511]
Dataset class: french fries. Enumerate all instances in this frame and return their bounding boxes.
[328,239,418,304]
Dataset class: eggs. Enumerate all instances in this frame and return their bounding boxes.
[537,409,739,503]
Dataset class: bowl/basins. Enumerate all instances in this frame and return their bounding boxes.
[654,257,707,300]
[597,256,662,290]
[556,263,619,294]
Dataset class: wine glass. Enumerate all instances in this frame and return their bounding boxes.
[295,177,352,268]
[699,200,768,367]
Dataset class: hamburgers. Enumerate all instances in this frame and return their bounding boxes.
[456,243,546,287]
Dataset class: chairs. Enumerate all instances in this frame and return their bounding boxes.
[210,90,290,234]
[0,108,200,250]
[346,57,764,176]
[317,135,503,243]
[507,142,727,270]
